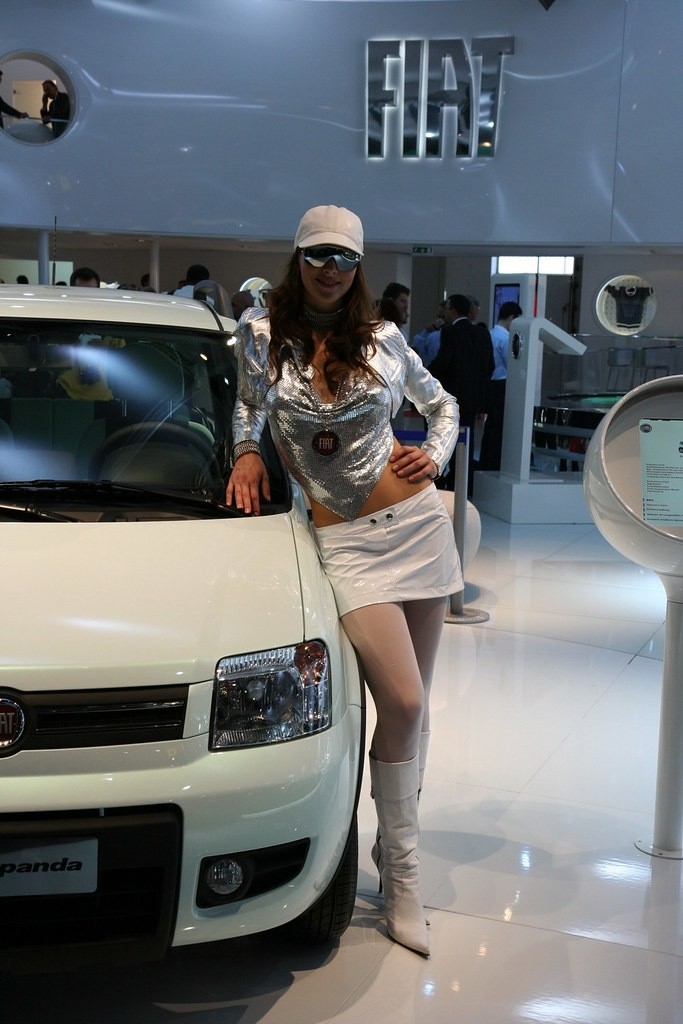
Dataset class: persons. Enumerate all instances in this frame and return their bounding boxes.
[0,263,522,516]
[226,205,460,954]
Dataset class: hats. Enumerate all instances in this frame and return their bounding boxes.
[293,205,365,256]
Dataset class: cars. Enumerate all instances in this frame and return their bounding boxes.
[0,278,368,979]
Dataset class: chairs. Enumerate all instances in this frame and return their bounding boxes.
[75,341,215,479]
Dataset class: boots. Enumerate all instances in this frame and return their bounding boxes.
[371,729,431,925]
[368,747,431,955]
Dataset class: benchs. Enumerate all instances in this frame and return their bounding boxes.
[0,398,53,451]
[53,399,206,457]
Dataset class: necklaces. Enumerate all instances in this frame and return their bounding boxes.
[305,364,345,456]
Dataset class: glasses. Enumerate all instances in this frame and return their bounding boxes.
[298,245,362,272]
